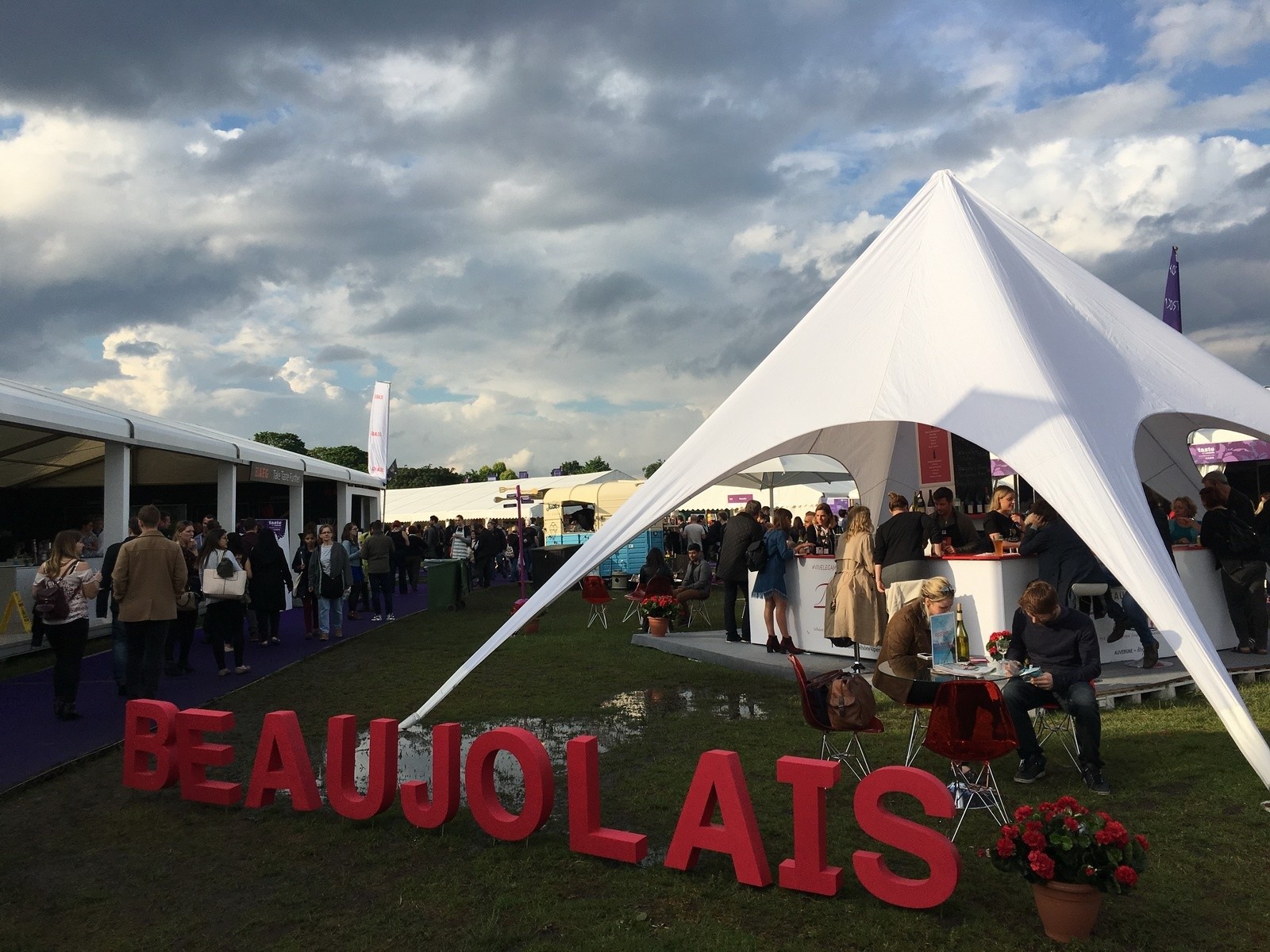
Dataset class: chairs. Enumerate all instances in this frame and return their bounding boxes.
[787,654,884,789]
[582,576,612,629]
[621,583,646,626]
[922,680,1020,843]
[1033,680,1097,773]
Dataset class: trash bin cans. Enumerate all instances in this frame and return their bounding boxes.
[421,557,471,612]
[611,571,627,589]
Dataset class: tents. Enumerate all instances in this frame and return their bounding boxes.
[401,170,1270,807]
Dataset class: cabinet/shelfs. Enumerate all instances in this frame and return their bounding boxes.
[531,544,582,584]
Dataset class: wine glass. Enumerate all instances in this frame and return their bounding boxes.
[673,572,677,580]
[92,568,105,590]
[996,640,1009,662]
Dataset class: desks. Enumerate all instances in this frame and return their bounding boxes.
[878,656,1041,777]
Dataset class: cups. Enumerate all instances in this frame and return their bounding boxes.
[41,554,47,561]
[993,535,1003,556]
[633,574,640,582]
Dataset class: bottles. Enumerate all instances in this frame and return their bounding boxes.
[342,588,350,600]
[824,528,832,555]
[955,602,969,662]
[815,530,824,555]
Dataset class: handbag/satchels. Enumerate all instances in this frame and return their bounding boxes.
[805,669,877,730]
[292,572,302,597]
[176,590,197,611]
[202,568,247,599]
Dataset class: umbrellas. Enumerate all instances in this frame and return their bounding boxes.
[713,454,854,525]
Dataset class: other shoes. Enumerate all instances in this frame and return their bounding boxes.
[386,613,395,621]
[1141,637,1160,669]
[218,668,230,676]
[235,665,250,674]
[305,628,343,641]
[348,612,364,621]
[54,697,83,721]
[727,634,751,644]
[1237,638,1268,655]
[1106,613,1131,644]
[370,614,382,622]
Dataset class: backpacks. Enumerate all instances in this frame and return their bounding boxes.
[505,528,534,559]
[31,560,85,622]
[745,540,768,572]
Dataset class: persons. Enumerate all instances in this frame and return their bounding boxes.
[486,517,544,581]
[569,504,595,530]
[875,491,943,593]
[823,506,887,645]
[1002,580,1111,794]
[30,504,189,723]
[983,485,1177,668]
[1168,469,1270,655]
[292,524,353,640]
[928,486,981,554]
[638,499,857,655]
[873,576,981,785]
[423,515,492,588]
[175,514,293,677]
[341,519,424,621]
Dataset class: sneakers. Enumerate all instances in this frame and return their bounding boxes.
[1013,763,1046,784]
[1080,767,1110,796]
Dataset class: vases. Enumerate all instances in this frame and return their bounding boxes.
[648,617,668,637]
[524,619,538,634]
[1032,881,1104,943]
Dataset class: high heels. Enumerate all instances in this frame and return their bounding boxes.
[780,636,806,654]
[766,635,781,653]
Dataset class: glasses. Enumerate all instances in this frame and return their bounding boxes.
[350,528,359,532]
[219,536,228,539]
[931,584,956,595]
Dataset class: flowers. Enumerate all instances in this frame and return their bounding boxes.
[642,595,684,619]
[978,796,1150,895]
[987,631,1012,660]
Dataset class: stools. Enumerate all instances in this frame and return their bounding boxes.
[1071,583,1109,620]
[688,598,712,628]
